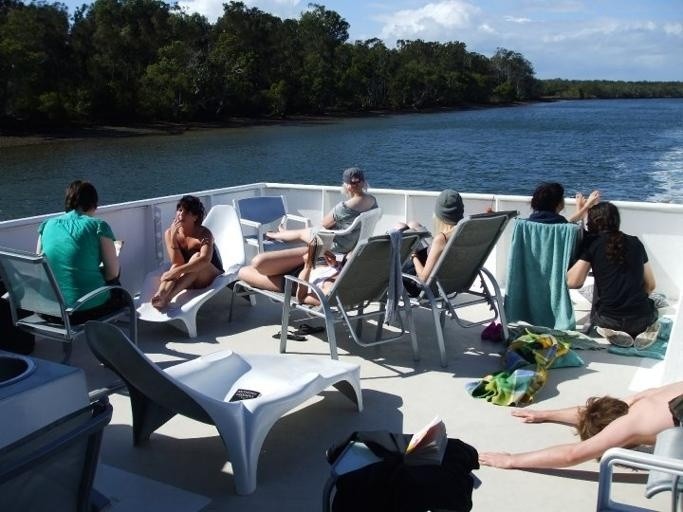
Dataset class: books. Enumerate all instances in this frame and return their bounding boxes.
[403,417,448,465]
[229,389,261,401]
[413,227,433,251]
[99,240,124,267]
[306,228,336,270]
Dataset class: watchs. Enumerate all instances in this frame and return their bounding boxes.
[410,255,418,262]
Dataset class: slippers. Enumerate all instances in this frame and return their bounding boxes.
[292,324,324,335]
[271,331,307,341]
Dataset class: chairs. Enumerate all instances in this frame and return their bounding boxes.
[401,210,520,368]
[0,245,139,364]
[304,208,383,262]
[232,194,311,253]
[85,319,364,494]
[597,428,681,512]
[136,204,255,339]
[280,231,420,361]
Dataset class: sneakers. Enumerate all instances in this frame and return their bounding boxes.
[481,321,504,343]
[635,324,661,350]
[596,326,633,346]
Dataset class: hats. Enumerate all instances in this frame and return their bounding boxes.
[434,188,463,225]
[342,168,365,185]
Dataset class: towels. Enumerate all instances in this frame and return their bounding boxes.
[504,218,578,334]
[605,317,669,360]
[506,319,606,351]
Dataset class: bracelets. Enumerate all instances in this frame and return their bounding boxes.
[331,261,342,273]
[170,246,180,250]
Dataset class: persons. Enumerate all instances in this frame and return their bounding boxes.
[566,191,662,350]
[478,383,683,469]
[35,181,129,323]
[375,190,463,298]
[529,181,600,223]
[237,222,408,308]
[151,196,224,310]
[266,167,377,253]
